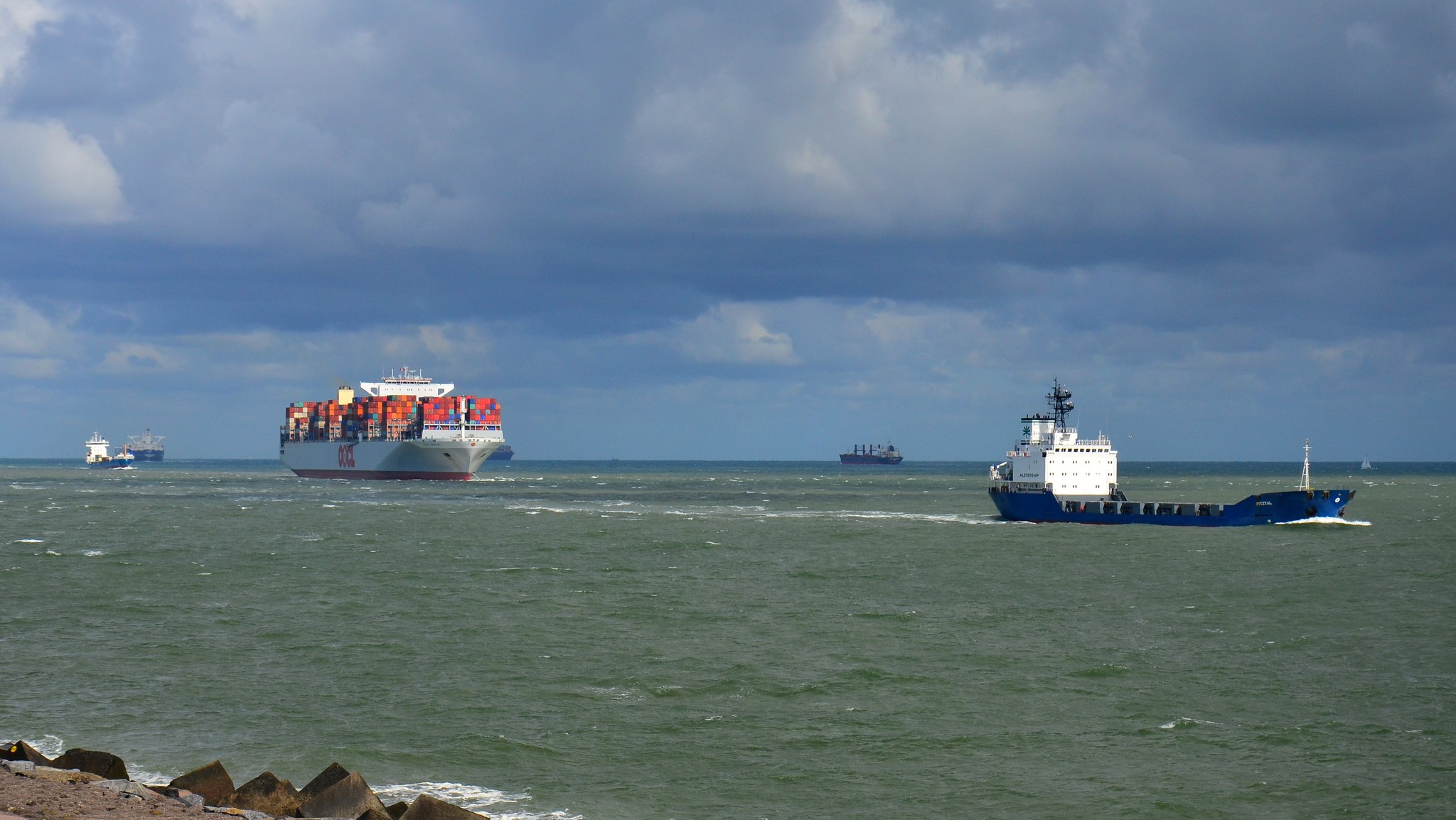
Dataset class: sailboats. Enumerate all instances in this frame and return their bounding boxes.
[1361,453,1378,470]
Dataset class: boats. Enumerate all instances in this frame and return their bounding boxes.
[839,437,904,465]
[279,366,506,481]
[987,376,1358,526]
[484,444,515,460]
[84,431,135,468]
[114,429,167,462]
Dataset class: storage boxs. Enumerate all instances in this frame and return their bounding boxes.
[285,394,503,440]
